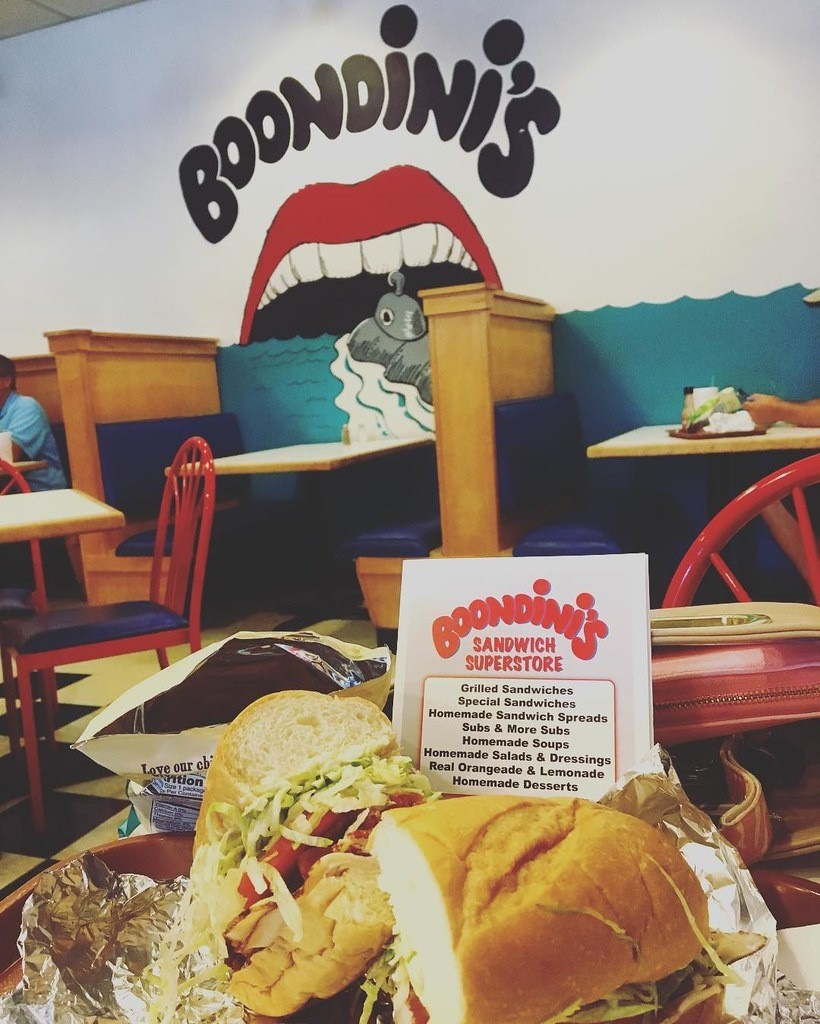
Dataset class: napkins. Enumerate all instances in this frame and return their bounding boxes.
[704,412,756,434]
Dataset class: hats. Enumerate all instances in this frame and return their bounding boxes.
[803,290,820,307]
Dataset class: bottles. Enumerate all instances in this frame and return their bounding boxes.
[682,386,694,432]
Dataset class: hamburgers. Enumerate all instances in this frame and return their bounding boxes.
[147,689,744,1024]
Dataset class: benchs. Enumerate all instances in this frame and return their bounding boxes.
[351,282,616,629]
[43,328,284,628]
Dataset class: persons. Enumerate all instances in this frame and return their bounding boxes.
[0,354,66,606]
[742,286,820,429]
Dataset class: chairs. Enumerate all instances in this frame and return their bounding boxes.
[0,437,214,835]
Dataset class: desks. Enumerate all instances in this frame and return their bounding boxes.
[586,423,820,607]
[1,489,125,546]
[165,437,429,603]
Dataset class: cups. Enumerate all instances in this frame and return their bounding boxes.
[693,387,719,413]
[0,431,14,466]
